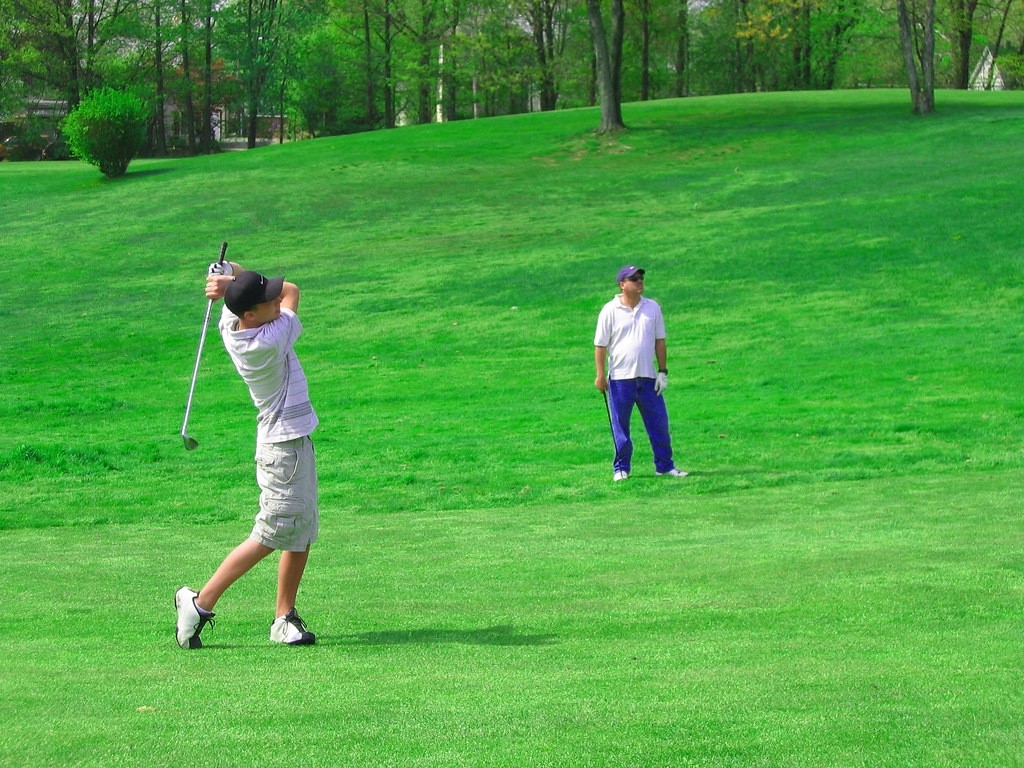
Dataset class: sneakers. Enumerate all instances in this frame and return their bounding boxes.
[614,471,627,481]
[656,468,689,477]
[270,607,315,645]
[174,586,216,649]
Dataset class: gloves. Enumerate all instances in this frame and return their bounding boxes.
[655,372,667,396]
[208,261,234,277]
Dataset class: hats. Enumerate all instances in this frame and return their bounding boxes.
[617,264,645,282]
[223,271,285,314]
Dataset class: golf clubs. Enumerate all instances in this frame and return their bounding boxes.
[181,240,229,451]
[601,389,631,481]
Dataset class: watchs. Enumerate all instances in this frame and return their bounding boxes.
[657,369,668,376]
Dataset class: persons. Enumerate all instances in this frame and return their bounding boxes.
[594,266,689,482]
[173,260,321,649]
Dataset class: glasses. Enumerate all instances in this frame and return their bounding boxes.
[625,275,644,282]
